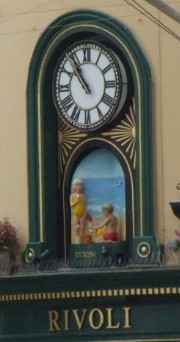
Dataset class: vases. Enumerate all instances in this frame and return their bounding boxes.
[0,250,17,276]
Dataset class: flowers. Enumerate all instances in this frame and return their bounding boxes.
[0,216,21,253]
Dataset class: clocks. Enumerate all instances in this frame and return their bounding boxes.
[51,39,133,133]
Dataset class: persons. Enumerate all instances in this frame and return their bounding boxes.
[88,203,122,243]
[69,179,88,244]
